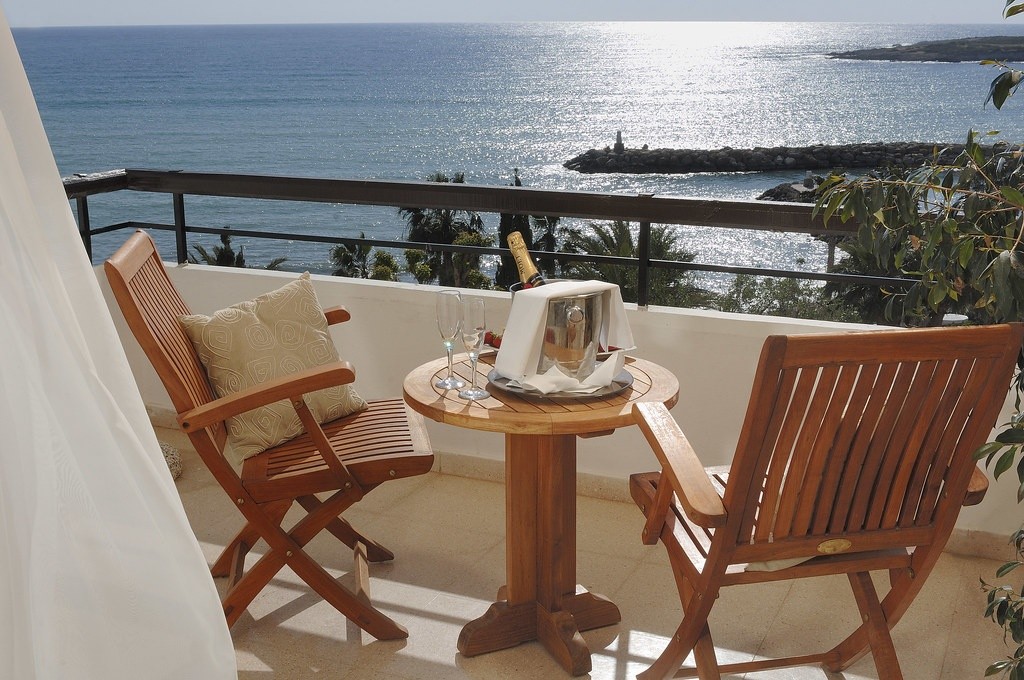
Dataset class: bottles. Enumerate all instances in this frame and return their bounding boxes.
[507,231,546,288]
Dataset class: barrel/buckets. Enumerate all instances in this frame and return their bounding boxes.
[509,279,605,382]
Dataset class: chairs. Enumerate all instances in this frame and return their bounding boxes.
[626,320,1024,679]
[104,227,433,641]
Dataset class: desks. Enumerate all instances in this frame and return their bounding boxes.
[400,343,680,675]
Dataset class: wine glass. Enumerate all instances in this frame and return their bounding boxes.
[458,299,490,401]
[435,290,466,390]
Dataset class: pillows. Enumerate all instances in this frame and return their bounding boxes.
[175,271,369,463]
